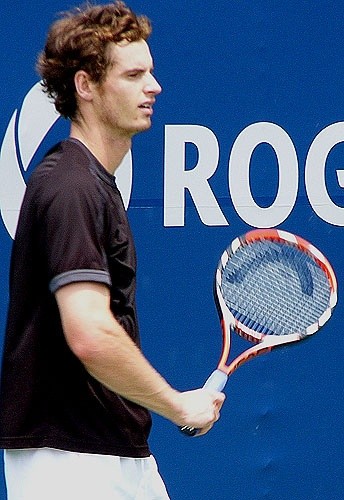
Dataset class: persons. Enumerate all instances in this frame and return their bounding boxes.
[0,1,227,500]
